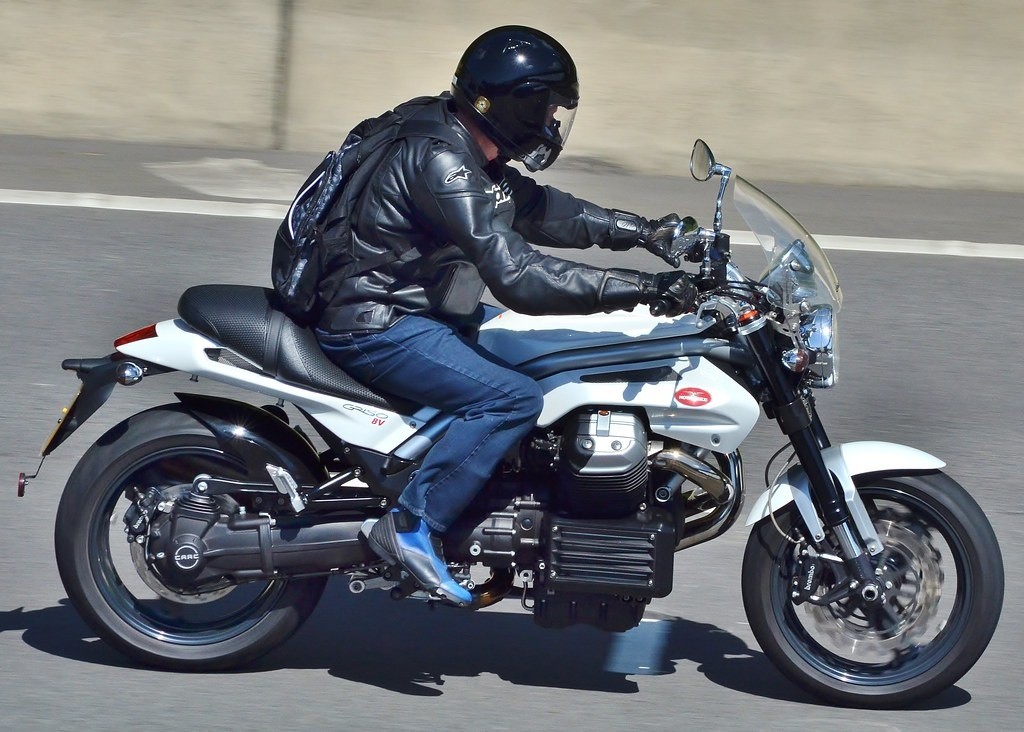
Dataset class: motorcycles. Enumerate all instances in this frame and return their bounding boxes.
[16,138,1007,713]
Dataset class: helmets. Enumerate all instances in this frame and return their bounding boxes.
[450,25,579,173]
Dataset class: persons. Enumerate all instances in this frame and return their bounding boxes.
[315,24,701,608]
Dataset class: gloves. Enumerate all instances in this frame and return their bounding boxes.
[605,208,706,269]
[597,267,698,317]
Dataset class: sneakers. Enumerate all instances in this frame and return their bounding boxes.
[367,504,472,609]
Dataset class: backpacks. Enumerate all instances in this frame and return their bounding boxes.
[271,96,483,314]
